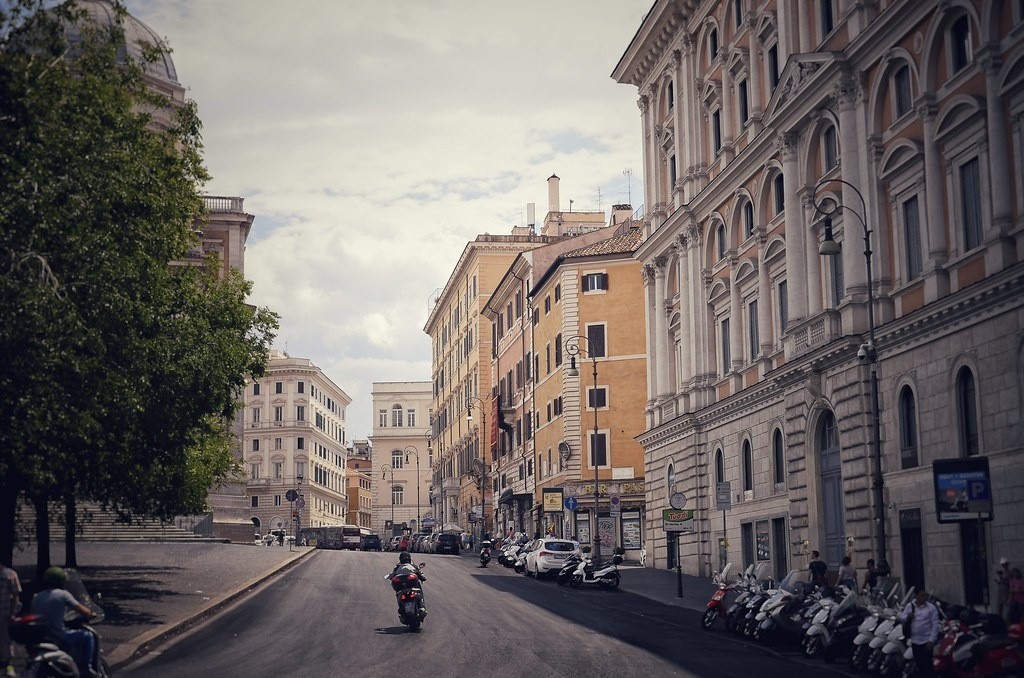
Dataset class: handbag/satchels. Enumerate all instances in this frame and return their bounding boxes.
[818,575,827,585]
[902,601,914,637]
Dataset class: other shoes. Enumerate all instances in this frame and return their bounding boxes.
[4,665,16,678]
[88,665,102,678]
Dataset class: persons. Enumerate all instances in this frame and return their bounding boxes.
[571,536,580,550]
[459,527,559,552]
[0,562,23,678]
[30,566,102,678]
[862,558,890,594]
[835,557,859,592]
[266,529,285,546]
[993,558,1024,625]
[900,590,940,678]
[807,550,829,586]
[388,552,427,615]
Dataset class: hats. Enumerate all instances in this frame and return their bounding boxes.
[1000,557,1009,564]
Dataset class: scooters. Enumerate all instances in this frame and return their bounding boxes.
[700,562,1024,678]
[640,540,646,567]
[479,538,494,567]
[569,555,623,589]
[557,546,625,585]
[384,562,427,633]
[497,533,536,573]
[9,569,113,678]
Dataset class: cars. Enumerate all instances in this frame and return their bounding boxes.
[524,538,576,579]
[384,533,459,555]
[362,534,383,551]
[255,534,295,546]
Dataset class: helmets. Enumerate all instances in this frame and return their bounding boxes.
[400,552,411,564]
[43,565,66,588]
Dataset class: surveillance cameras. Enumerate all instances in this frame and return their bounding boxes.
[857,349,867,360]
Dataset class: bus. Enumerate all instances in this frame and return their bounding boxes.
[356,526,373,548]
[295,525,361,550]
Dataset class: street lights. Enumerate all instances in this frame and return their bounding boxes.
[381,464,393,521]
[404,445,420,533]
[566,336,602,565]
[296,473,303,546]
[425,429,443,532]
[465,397,486,547]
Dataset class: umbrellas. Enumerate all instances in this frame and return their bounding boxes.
[439,520,463,532]
